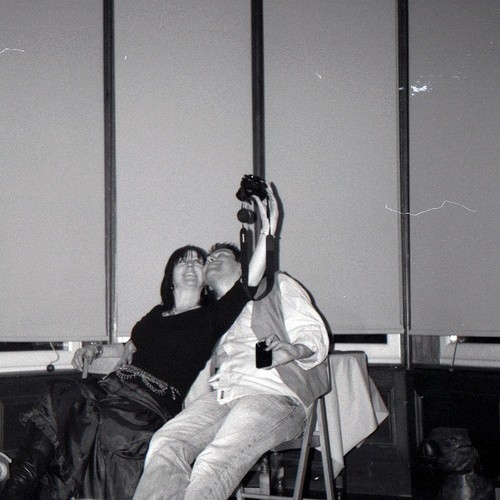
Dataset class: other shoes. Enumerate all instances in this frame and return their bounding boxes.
[0,456,44,500]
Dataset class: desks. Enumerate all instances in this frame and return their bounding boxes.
[275,351,393,500]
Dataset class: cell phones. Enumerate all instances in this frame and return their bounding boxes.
[256,341,272,368]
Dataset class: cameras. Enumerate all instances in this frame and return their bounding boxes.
[236,174,269,205]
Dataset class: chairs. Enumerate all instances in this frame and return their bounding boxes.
[83,354,123,379]
[229,395,337,500]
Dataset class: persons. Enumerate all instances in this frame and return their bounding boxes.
[50,179,280,499]
[124,240,336,500]
